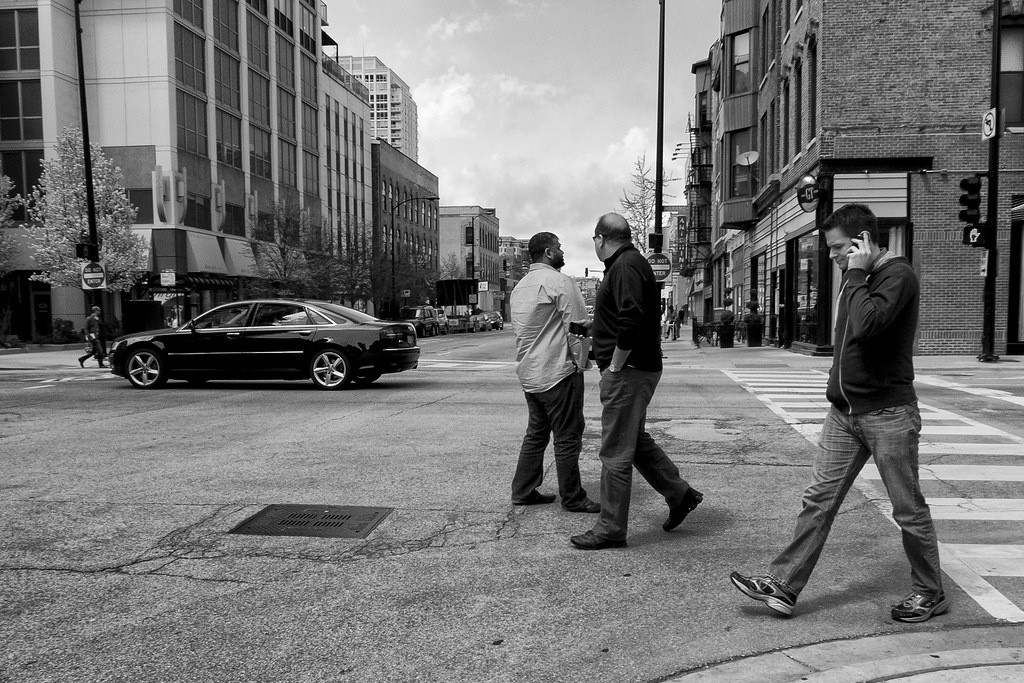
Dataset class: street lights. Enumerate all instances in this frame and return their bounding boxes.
[472,211,493,314]
[391,195,440,297]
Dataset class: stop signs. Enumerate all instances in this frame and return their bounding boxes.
[81,262,107,289]
[644,252,672,282]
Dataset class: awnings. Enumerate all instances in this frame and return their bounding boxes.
[188,231,313,282]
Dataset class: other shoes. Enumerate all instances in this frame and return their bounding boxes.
[78,358,84,368]
[665,336,680,338]
[99,364,108,368]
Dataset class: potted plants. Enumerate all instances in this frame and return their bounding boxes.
[717,287,736,348]
[743,289,765,347]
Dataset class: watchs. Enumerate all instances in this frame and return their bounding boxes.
[609,364,621,372]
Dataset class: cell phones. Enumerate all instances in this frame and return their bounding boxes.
[569,322,588,338]
[848,234,864,253]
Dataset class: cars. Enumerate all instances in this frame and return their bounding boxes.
[470,315,492,332]
[451,316,476,334]
[481,311,503,330]
[107,298,421,392]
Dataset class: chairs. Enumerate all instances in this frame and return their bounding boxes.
[692,315,715,348]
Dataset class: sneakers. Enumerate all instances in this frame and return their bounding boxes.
[512,490,557,504]
[891,590,948,622]
[570,530,628,549]
[663,486,704,530]
[558,496,601,513]
[730,571,797,614]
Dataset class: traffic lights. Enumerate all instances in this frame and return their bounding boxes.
[963,224,984,245]
[959,178,980,224]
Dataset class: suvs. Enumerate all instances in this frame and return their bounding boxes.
[397,305,436,337]
[433,309,449,335]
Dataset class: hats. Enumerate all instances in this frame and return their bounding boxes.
[668,305,673,308]
[92,306,101,311]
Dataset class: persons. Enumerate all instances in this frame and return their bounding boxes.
[729,203,948,623]
[570,212,704,553]
[78,306,109,368]
[661,304,686,340]
[510,233,602,513]
